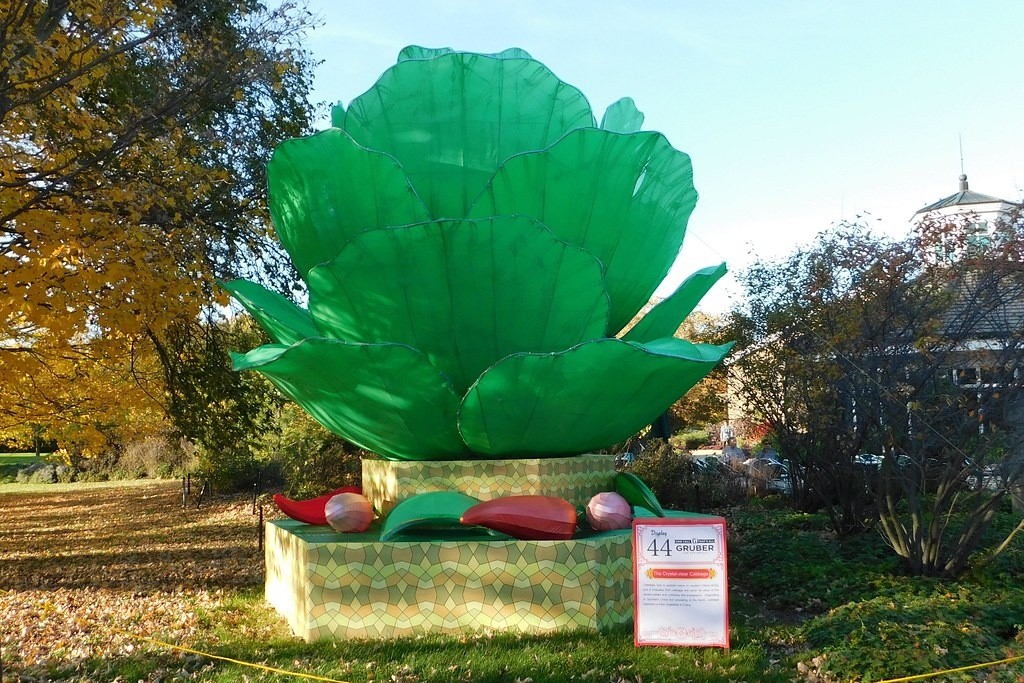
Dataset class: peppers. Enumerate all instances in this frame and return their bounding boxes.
[272,467,667,542]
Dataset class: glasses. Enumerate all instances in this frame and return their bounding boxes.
[765,443,771,445]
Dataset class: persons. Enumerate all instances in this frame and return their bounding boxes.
[720,420,733,448]
[724,437,746,461]
[757,438,775,460]
